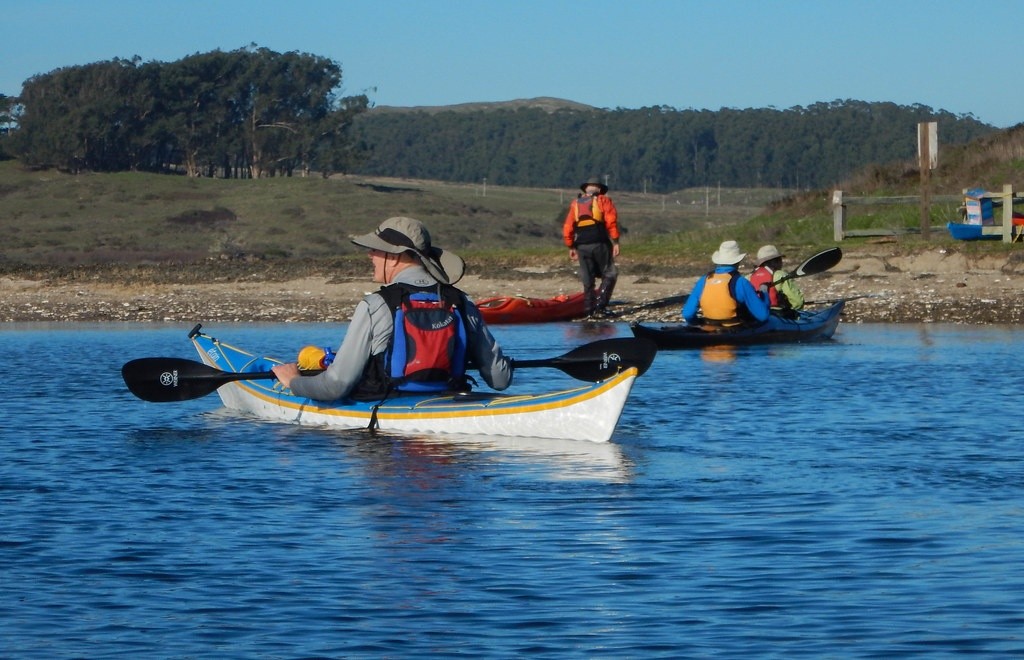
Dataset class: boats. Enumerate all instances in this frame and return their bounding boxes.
[630,300,845,350]
[474,288,600,325]
[947,222,1024,242]
[189,323,637,444]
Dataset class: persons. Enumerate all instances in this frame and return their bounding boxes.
[682,238,800,338]
[560,175,625,325]
[269,216,513,409]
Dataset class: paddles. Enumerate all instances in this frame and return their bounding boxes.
[121,335,658,403]
[769,244,843,291]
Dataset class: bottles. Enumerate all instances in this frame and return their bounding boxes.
[323,346,335,366]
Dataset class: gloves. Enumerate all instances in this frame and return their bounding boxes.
[760,281,771,292]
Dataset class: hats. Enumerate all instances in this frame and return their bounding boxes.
[580,176,609,195]
[712,240,747,265]
[348,217,465,287]
[754,244,786,266]
